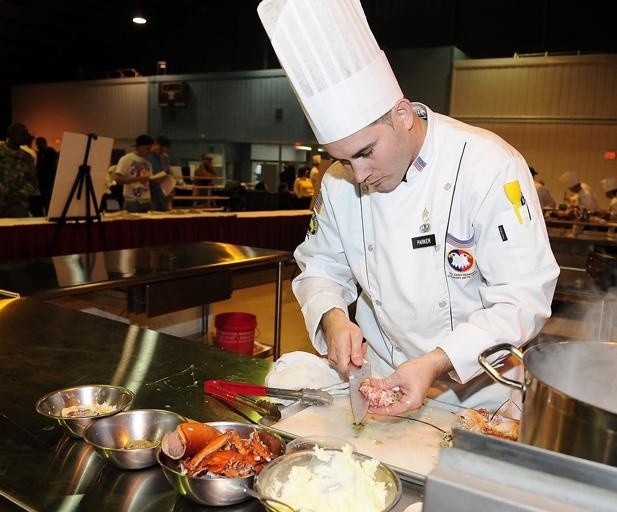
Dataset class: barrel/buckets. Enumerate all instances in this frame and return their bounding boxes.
[214,312,260,357]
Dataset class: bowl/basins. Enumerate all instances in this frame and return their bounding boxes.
[283,436,360,456]
[36,384,135,440]
[159,420,285,503]
[257,451,401,512]
[84,409,186,470]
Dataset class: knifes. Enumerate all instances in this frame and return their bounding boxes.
[346,335,374,426]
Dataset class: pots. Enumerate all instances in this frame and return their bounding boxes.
[477,340,616,466]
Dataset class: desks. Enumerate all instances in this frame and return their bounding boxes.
[1,240,297,364]
[0,205,314,281]
[160,185,315,212]
[0,294,413,512]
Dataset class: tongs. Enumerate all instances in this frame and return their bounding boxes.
[203,379,334,423]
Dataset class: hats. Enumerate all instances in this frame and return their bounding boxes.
[130,134,154,147]
[256,0,405,146]
[559,171,580,189]
[600,176,617,193]
[528,167,538,176]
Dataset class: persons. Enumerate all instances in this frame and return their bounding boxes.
[292,97,561,418]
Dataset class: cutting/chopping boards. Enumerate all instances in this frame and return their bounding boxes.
[265,391,522,490]
